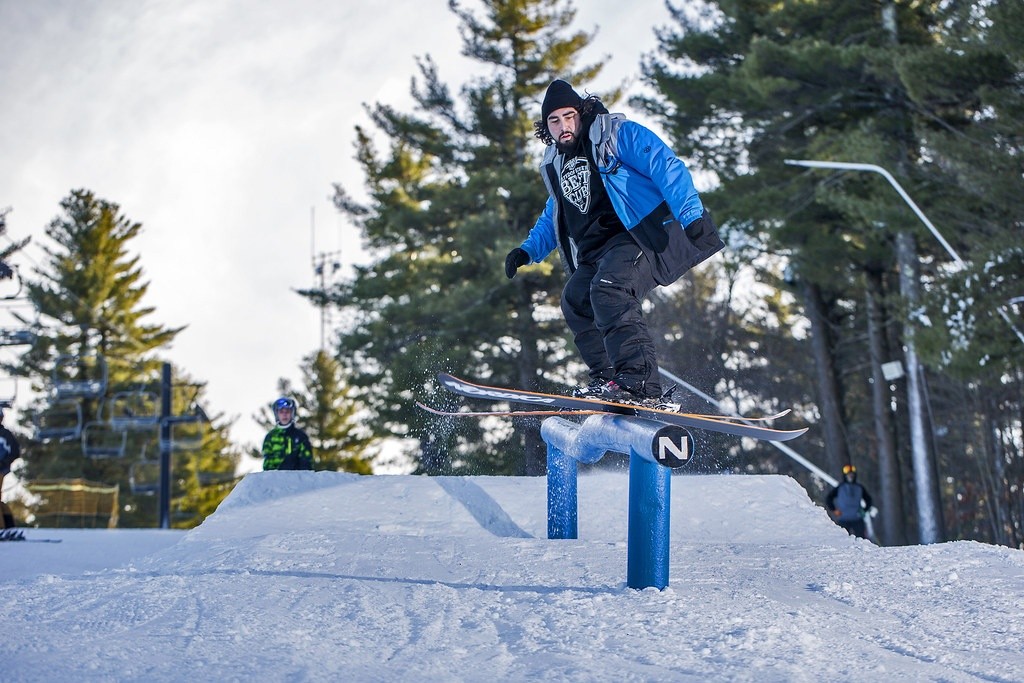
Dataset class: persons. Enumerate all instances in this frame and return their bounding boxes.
[262,398,313,471]
[825,465,872,539]
[505,81,726,406]
[0,410,26,541]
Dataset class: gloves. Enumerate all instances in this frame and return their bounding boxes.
[505,248,530,279]
[685,218,717,252]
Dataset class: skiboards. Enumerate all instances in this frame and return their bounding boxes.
[414,370,810,441]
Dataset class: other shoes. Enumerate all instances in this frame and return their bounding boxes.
[566,377,612,399]
[586,380,664,408]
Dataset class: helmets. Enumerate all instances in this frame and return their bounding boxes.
[843,464,857,474]
[273,398,297,420]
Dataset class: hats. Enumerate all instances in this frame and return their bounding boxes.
[541,80,584,123]
